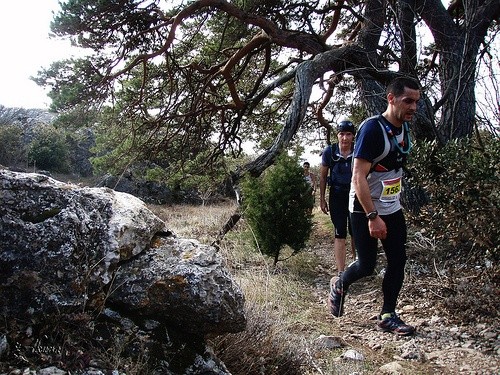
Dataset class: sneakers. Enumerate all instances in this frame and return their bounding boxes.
[328,276,348,318]
[377,312,414,334]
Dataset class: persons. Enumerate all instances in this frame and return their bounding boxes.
[301,161,316,203]
[320,120,358,276]
[328,76,420,336]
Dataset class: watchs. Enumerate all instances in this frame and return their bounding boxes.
[366,210,378,220]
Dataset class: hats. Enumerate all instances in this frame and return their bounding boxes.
[337,121,356,136]
